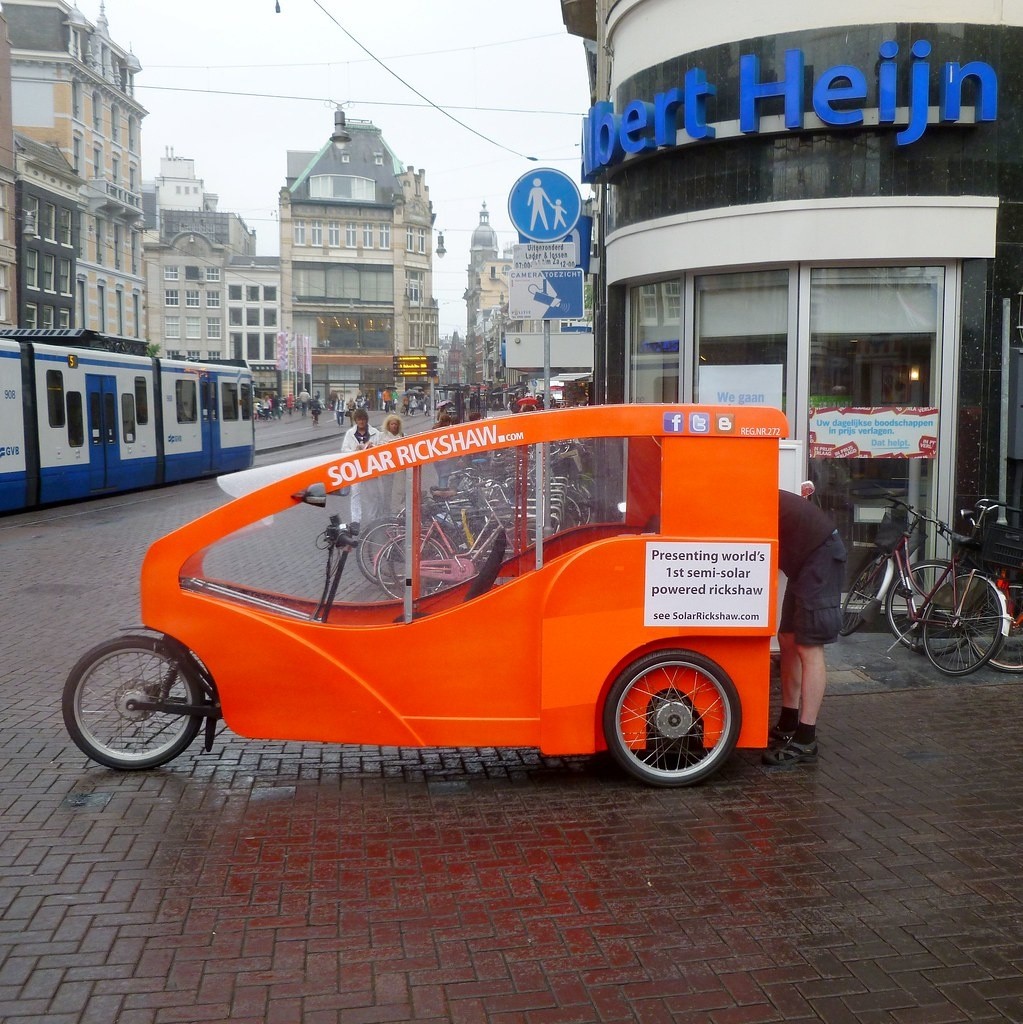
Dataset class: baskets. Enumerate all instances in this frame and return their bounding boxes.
[551,456,579,478]
[875,512,928,559]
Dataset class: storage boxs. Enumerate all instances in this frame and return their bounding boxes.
[980,522,1023,570]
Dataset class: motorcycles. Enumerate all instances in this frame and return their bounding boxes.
[61,402,790,790]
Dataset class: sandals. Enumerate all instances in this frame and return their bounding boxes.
[761,737,817,766]
[768,719,799,743]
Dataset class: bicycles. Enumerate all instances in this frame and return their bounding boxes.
[837,495,1015,676]
[355,443,591,601]
[884,504,1023,674]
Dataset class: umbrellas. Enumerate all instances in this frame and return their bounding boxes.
[385,387,397,390]
[517,398,539,406]
[436,400,452,409]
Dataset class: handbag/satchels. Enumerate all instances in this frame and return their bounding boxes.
[334,413,337,421]
[345,411,351,416]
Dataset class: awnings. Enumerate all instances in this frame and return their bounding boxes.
[537,373,591,382]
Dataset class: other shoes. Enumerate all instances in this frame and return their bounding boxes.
[348,521,361,530]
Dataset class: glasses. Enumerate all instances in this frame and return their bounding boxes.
[360,436,364,443]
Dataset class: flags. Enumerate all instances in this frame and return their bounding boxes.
[275,332,312,374]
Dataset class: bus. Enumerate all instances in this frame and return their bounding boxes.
[0,328,255,519]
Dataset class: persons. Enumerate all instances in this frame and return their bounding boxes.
[341,408,407,552]
[761,488,848,766]
[432,406,481,429]
[252,388,322,424]
[382,387,431,416]
[325,388,372,427]
[507,393,556,413]
[558,396,590,408]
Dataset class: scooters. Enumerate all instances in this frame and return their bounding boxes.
[311,408,320,426]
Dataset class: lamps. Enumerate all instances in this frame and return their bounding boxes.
[329,106,351,151]
[23,213,37,242]
[436,231,447,259]
